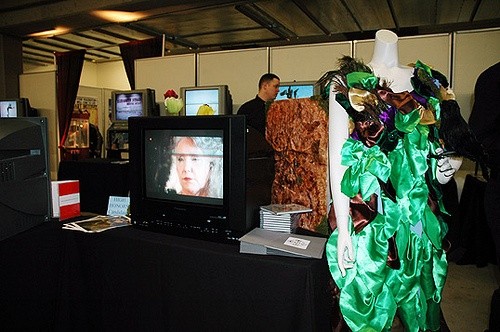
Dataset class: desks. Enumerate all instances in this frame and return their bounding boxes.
[0,214,342,332]
[58,160,138,216]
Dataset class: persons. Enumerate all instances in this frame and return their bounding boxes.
[236,73,280,138]
[324,29,463,332]
[163,137,222,198]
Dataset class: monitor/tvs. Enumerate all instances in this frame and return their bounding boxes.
[180,85,233,116]
[273,80,321,102]
[0,117,53,241]
[127,116,275,245]
[0,97,38,118]
[111,89,156,122]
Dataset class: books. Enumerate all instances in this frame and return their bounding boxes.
[259,204,314,216]
